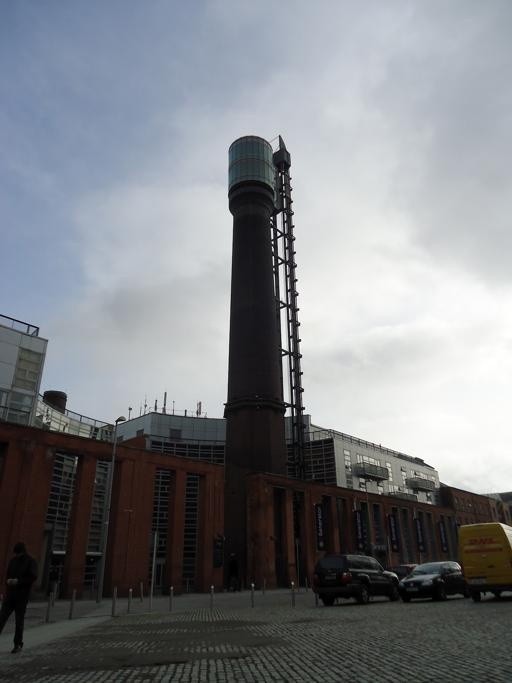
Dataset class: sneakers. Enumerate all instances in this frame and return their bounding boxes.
[11,641,25,654]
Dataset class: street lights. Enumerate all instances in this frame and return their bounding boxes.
[95,417,126,602]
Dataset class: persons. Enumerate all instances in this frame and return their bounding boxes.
[1,542,35,654]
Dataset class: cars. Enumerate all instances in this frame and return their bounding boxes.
[312,554,462,605]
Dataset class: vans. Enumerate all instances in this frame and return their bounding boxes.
[457,522,512,602]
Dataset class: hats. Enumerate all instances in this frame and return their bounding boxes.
[13,541,27,552]
